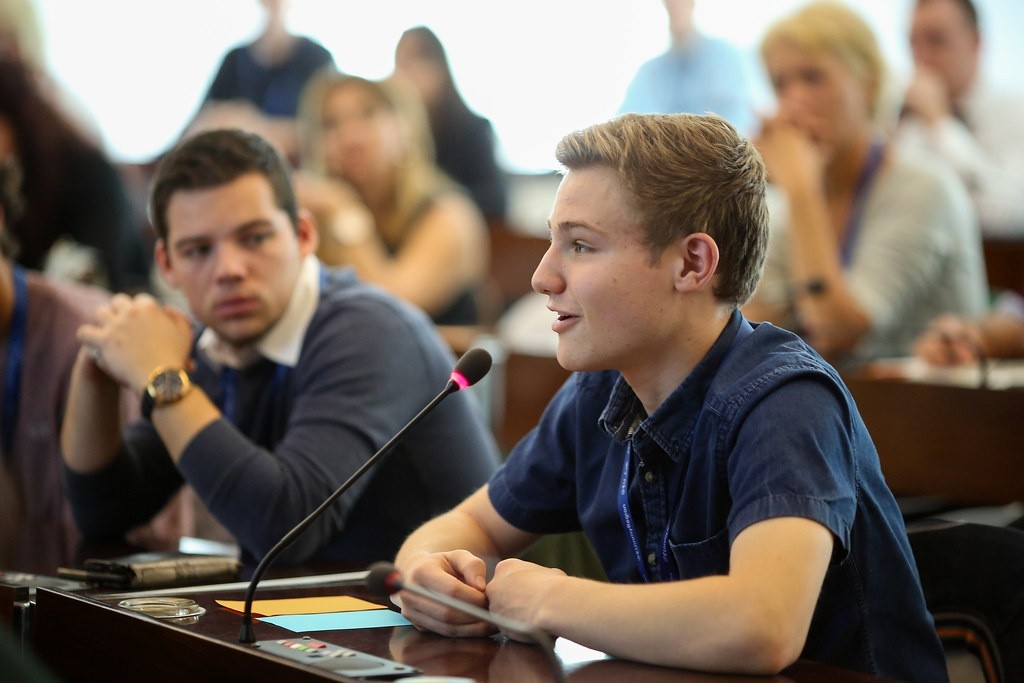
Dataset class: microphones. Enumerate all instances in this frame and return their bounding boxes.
[239,347,493,644]
[363,561,568,683]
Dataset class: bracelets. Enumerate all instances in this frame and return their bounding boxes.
[792,269,849,304]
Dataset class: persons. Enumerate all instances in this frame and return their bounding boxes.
[895,0,1024,243]
[5,255,182,570]
[390,114,941,683]
[621,0,777,152]
[383,23,505,220]
[164,0,344,159]
[2,41,155,290]
[287,58,484,325]
[720,3,986,360]
[914,296,1024,368]
[57,130,510,590]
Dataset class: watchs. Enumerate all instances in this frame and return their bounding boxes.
[138,365,195,422]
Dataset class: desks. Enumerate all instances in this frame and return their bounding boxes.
[0,566,717,683]
[503,352,1024,517]
